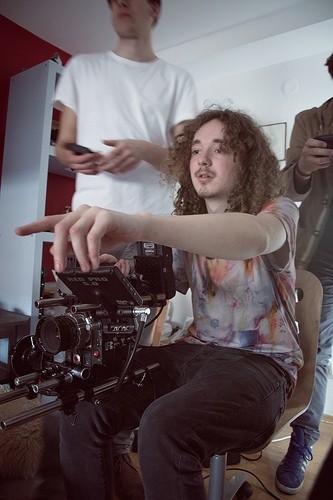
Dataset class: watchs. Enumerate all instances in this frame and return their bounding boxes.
[297,164,312,177]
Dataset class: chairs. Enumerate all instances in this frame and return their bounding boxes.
[137,302,169,349]
[208,271,324,499]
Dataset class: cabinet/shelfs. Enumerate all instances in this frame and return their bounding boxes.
[0,60,77,367]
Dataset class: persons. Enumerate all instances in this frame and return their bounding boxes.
[276,53,333,494]
[52,0,199,500]
[15,103,303,500]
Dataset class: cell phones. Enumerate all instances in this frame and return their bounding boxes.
[314,133,333,150]
[64,142,91,154]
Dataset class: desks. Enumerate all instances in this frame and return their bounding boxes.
[0,306,31,391]
